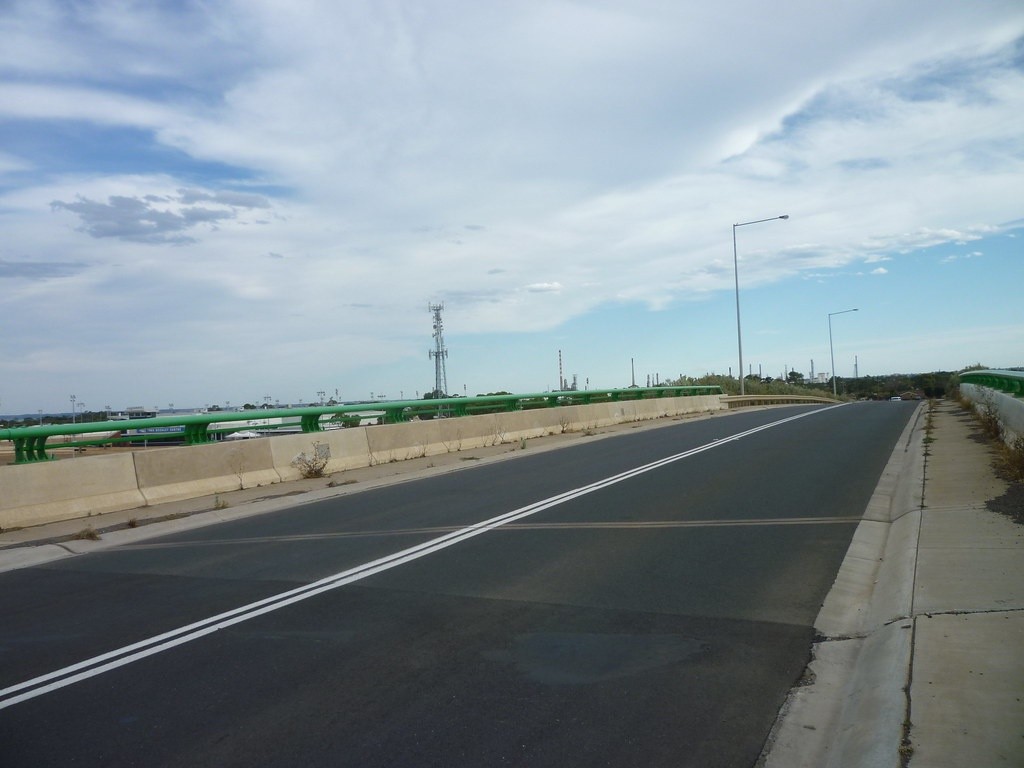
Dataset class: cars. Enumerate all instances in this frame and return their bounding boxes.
[890,396,901,401]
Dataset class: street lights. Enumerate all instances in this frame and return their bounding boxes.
[316,390,326,419]
[69,395,76,423]
[78,403,86,422]
[827,308,859,396]
[733,214,790,396]
[38,409,43,427]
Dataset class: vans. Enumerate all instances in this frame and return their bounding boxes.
[911,394,921,400]
